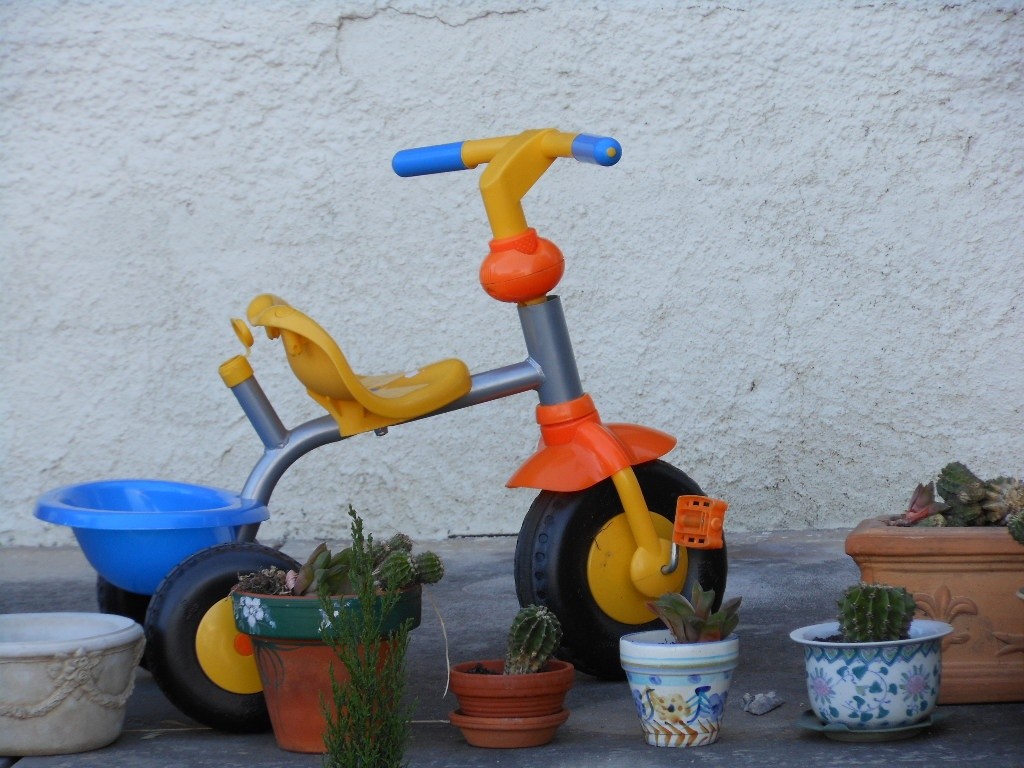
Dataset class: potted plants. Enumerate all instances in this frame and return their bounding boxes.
[232,531,444,754]
[845,461,1024,706]
[789,580,955,740]
[447,603,575,749]
[619,581,743,746]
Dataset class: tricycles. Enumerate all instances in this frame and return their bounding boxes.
[27,127,729,737]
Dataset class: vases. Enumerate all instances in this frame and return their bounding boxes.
[0,612,145,756]
